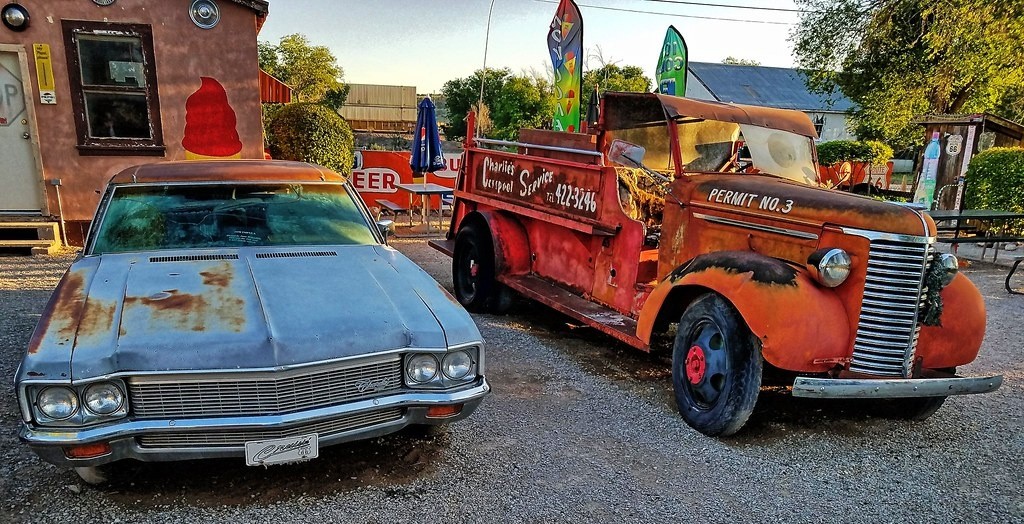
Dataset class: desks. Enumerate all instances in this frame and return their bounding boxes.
[924,210,1024,262]
[395,183,455,239]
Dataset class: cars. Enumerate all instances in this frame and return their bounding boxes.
[12,159,492,487]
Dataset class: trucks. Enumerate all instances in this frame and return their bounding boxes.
[428,92,1003,440]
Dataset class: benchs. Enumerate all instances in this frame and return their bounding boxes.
[934,225,979,252]
[373,200,411,228]
[442,196,455,230]
[935,236,1024,262]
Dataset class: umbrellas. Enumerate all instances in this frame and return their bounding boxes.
[409,97,445,225]
